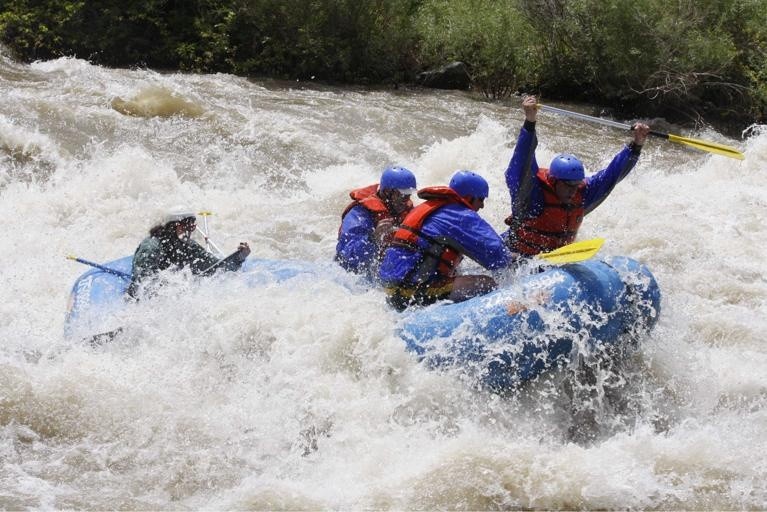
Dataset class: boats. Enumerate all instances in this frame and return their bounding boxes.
[62,254,660,399]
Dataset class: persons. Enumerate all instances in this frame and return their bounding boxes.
[502,95,650,273]
[376,170,525,314]
[128,204,249,303]
[334,166,417,274]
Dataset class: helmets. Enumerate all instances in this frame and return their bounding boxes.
[165,204,196,225]
[380,166,417,199]
[548,154,585,187]
[449,170,488,201]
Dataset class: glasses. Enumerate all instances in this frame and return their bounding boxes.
[179,223,197,232]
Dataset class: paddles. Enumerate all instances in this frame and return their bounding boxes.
[520,238,605,264]
[530,103,747,161]
